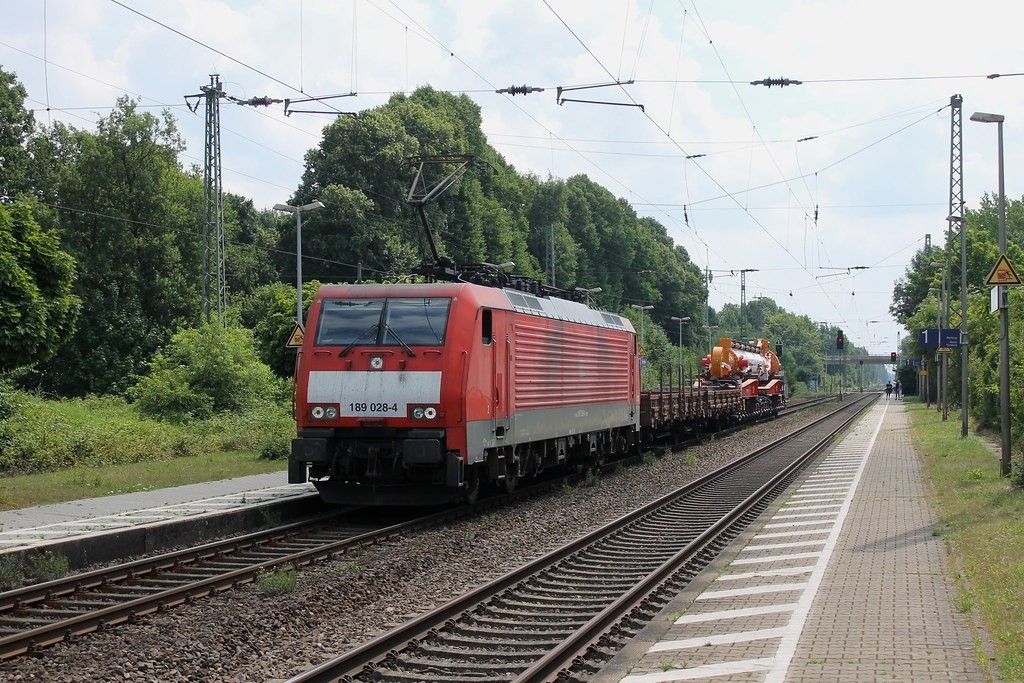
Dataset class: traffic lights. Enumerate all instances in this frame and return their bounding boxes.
[836,331,844,350]
[775,344,783,358]
[891,352,897,362]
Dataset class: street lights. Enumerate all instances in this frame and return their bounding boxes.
[631,304,654,392]
[671,316,691,393]
[931,261,949,421]
[946,214,969,438]
[702,325,719,356]
[273,202,326,384]
[970,112,1012,479]
[929,286,940,411]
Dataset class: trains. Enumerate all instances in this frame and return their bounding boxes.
[287,154,789,514]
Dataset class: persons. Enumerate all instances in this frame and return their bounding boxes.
[886,381,892,400]
[895,380,903,400]
